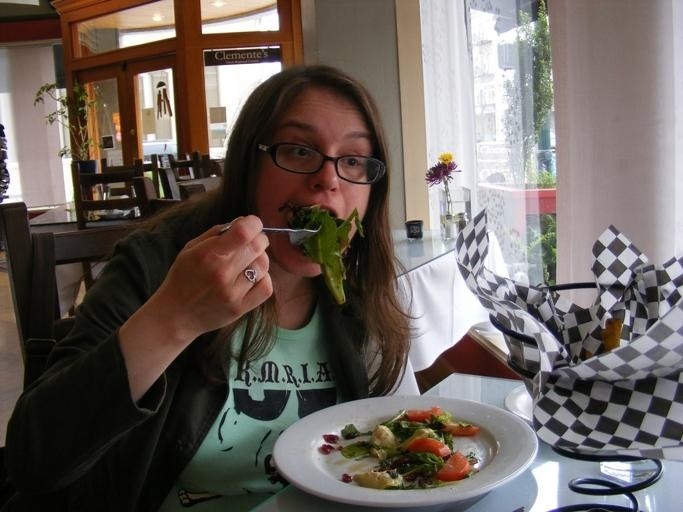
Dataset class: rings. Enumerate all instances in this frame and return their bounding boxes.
[243,266,258,283]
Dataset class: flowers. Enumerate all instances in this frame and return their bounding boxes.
[425,152,462,214]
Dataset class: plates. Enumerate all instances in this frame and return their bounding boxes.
[273,395,540,510]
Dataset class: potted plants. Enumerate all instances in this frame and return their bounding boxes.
[33,80,106,174]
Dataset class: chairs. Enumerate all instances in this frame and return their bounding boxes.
[0,200,146,384]
[70,150,218,217]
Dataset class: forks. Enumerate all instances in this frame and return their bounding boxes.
[217,222,328,246]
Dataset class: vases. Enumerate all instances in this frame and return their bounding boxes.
[439,215,465,239]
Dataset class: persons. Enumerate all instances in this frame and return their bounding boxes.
[6,67,422,512]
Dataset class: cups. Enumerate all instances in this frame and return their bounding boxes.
[405,220,424,238]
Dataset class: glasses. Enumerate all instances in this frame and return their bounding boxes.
[258,141,385,184]
